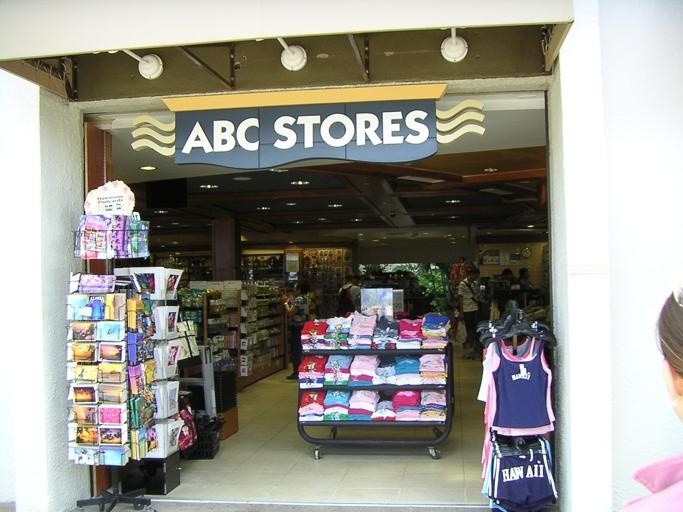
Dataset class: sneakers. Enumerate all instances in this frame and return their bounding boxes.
[287,374,298,379]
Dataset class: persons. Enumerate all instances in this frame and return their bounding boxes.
[501,268,518,286]
[337,274,361,317]
[459,266,488,348]
[623,288,683,512]
[517,267,531,289]
[284,279,309,379]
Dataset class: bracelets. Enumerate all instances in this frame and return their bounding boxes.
[284,302,288,306]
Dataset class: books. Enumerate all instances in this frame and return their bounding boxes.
[73,213,152,261]
[68,266,199,466]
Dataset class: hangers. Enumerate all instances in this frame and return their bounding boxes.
[476,300,557,348]
[489,427,547,458]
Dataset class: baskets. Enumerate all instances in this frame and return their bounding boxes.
[181,432,220,459]
[189,372,236,413]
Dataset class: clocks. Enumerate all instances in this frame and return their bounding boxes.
[521,247,532,258]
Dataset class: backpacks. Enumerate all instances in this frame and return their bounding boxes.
[339,285,355,312]
